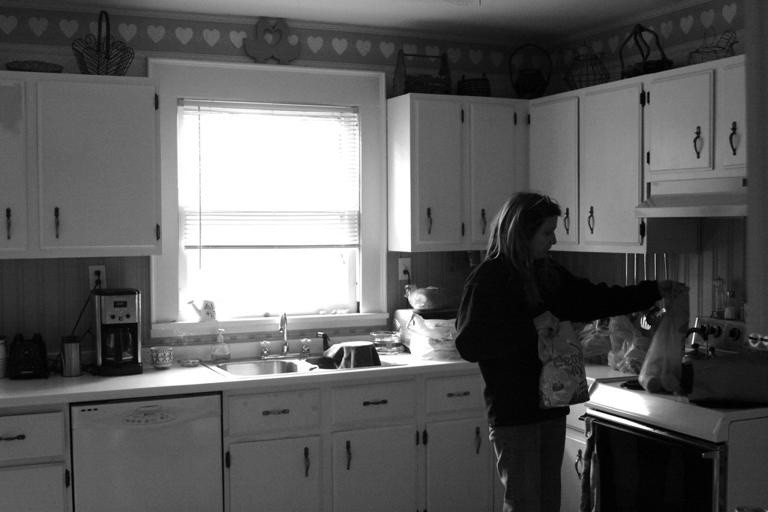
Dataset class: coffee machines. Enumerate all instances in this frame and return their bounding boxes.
[94,287,143,374]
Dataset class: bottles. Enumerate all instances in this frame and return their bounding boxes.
[712,277,738,319]
[60,334,81,377]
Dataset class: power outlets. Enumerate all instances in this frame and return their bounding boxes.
[88,265,107,291]
[398,257,411,280]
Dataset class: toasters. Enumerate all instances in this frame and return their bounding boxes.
[8,331,50,380]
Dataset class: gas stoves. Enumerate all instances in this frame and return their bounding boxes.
[597,373,768,415]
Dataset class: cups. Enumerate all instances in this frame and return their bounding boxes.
[149,346,172,368]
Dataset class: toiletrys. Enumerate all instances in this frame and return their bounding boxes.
[211,329,231,363]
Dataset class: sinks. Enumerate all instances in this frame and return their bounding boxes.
[306,355,404,369]
[214,362,303,377]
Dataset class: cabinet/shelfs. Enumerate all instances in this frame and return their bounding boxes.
[0,403,74,512]
[224,372,505,512]
[388,93,528,253]
[1,70,161,260]
[528,78,700,254]
[644,55,746,183]
[560,402,587,512]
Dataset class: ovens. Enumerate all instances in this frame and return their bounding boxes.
[579,406,730,512]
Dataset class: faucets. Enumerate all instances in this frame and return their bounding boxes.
[278,313,289,352]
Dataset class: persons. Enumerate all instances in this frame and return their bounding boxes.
[455,192,690,512]
